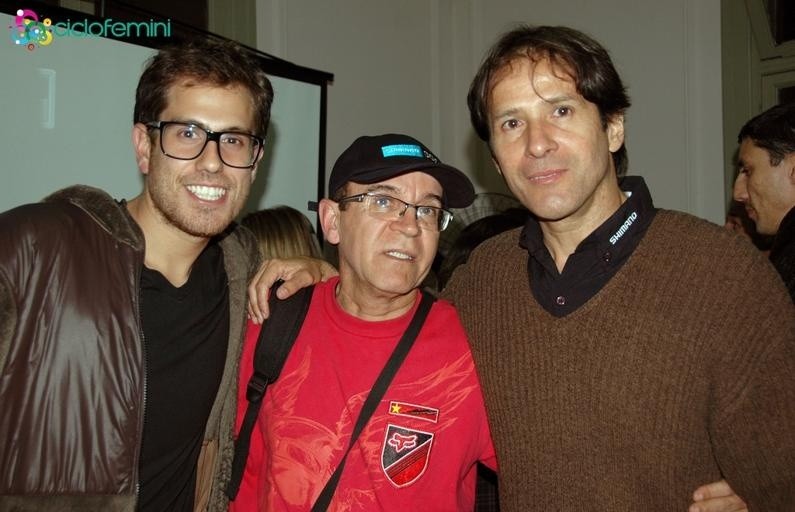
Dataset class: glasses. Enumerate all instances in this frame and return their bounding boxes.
[339,192,455,233]
[145,118,265,168]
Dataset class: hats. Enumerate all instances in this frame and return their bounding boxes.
[307,133,476,211]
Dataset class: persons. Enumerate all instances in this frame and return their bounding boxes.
[227,131,753,510]
[235,202,325,269]
[731,102,794,300]
[723,200,774,259]
[0,34,276,509]
[245,23,793,512]
